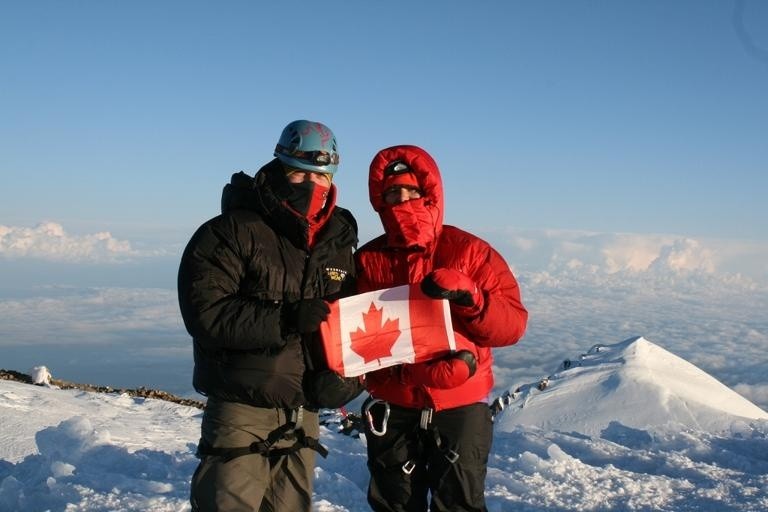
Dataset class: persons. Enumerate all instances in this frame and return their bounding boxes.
[178,119,359,511]
[357,142,526,510]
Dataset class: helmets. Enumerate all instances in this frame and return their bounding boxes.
[382,161,420,196]
[273,120,339,173]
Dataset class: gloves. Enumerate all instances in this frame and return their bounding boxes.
[398,350,477,389]
[421,267,484,319]
[305,370,363,410]
[281,298,330,335]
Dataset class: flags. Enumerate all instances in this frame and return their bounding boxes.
[318,285,456,377]
